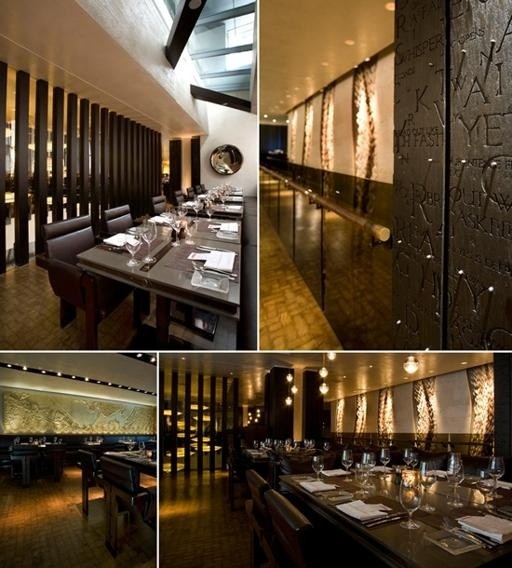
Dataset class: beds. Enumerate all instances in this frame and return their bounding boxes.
[276,467,512,568]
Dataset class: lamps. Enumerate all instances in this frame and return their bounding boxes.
[318,354,337,395]
[401,352,420,377]
[285,354,298,406]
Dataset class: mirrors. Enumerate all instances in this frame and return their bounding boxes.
[210,144,243,175]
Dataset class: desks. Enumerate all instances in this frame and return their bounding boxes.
[75,186,244,350]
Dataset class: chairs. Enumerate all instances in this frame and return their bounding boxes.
[413,447,448,471]
[263,488,314,568]
[1,459,15,479]
[35,184,206,350]
[463,454,491,476]
[244,469,270,568]
[77,449,147,556]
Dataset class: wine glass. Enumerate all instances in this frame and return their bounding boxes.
[127,441,153,462]
[126,184,233,267]
[254,438,329,458]
[312,448,504,529]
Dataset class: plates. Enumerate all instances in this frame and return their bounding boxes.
[423,526,482,555]
[290,476,315,483]
[188,230,239,294]
